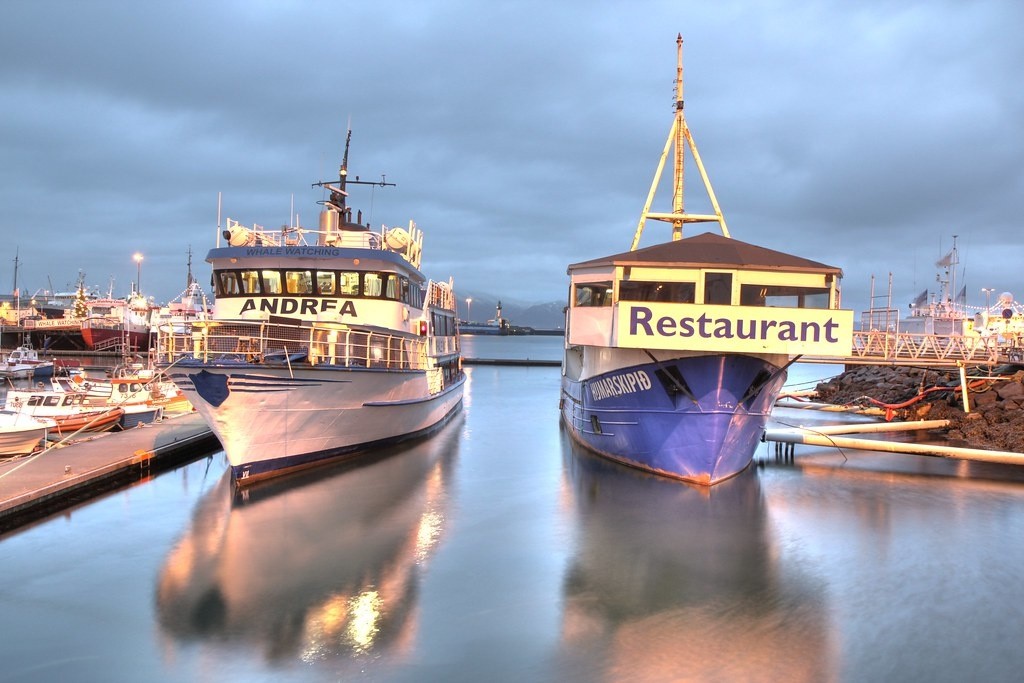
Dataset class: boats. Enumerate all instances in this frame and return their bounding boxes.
[155,281,212,357]
[0,342,55,383]
[899,233,1024,349]
[79,275,153,350]
[49,265,99,317]
[47,376,165,429]
[556,31,854,490]
[0,411,56,459]
[7,390,125,434]
[154,121,468,489]
[160,395,197,414]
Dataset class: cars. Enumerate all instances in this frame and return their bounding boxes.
[47,300,64,307]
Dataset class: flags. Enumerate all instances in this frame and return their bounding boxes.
[13,289,18,297]
[937,255,951,267]
[44,337,51,349]
[33,366,54,384]
[916,289,927,303]
[957,284,966,296]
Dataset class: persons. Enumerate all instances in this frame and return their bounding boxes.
[55,366,70,377]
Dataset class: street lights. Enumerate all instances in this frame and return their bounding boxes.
[980,287,996,316]
[133,251,143,295]
[465,298,472,322]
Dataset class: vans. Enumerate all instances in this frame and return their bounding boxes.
[19,315,42,326]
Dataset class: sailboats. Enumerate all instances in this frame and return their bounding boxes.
[106,315,168,382]
